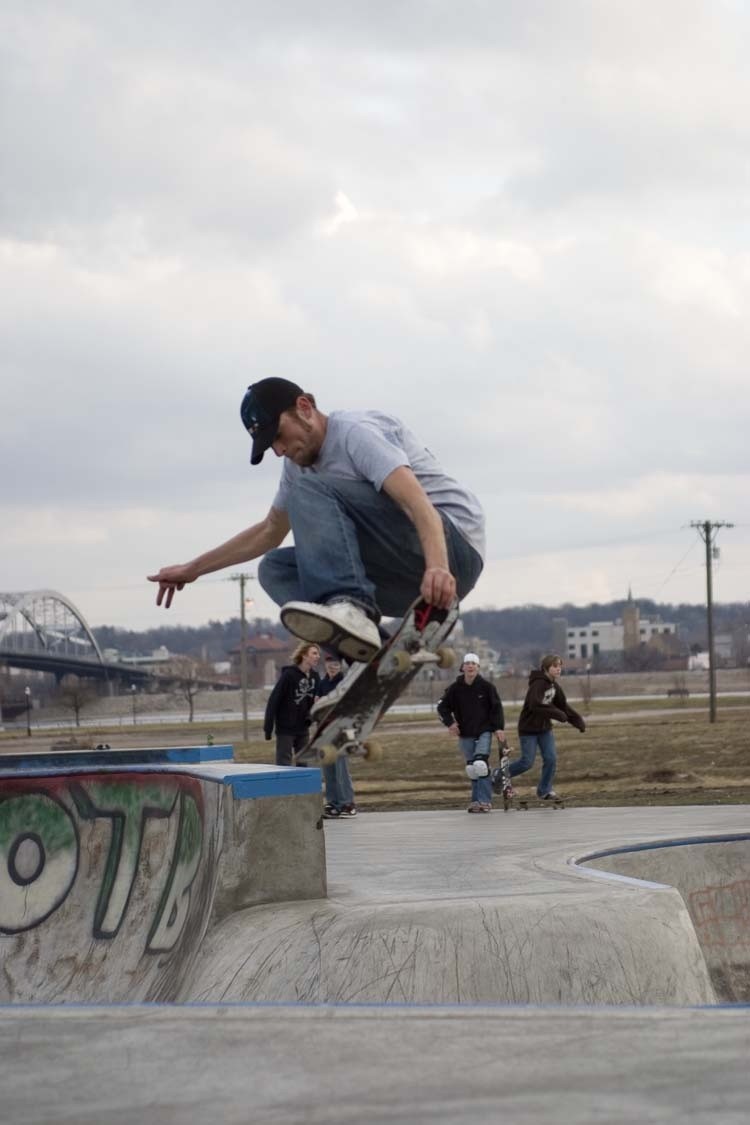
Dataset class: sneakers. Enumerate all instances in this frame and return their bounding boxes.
[280,601,381,663]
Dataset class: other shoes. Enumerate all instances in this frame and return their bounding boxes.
[322,803,357,818]
[492,768,504,795]
[480,802,493,813]
[467,802,480,813]
[538,791,563,802]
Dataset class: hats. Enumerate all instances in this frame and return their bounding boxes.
[324,651,334,660]
[241,377,304,465]
[459,653,480,672]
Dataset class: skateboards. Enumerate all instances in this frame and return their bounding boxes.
[293,591,468,766]
[497,738,519,812]
[511,795,577,811]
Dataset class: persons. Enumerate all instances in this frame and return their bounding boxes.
[436,650,509,810]
[490,655,586,801]
[315,651,356,819]
[145,377,490,724]
[261,638,322,768]
